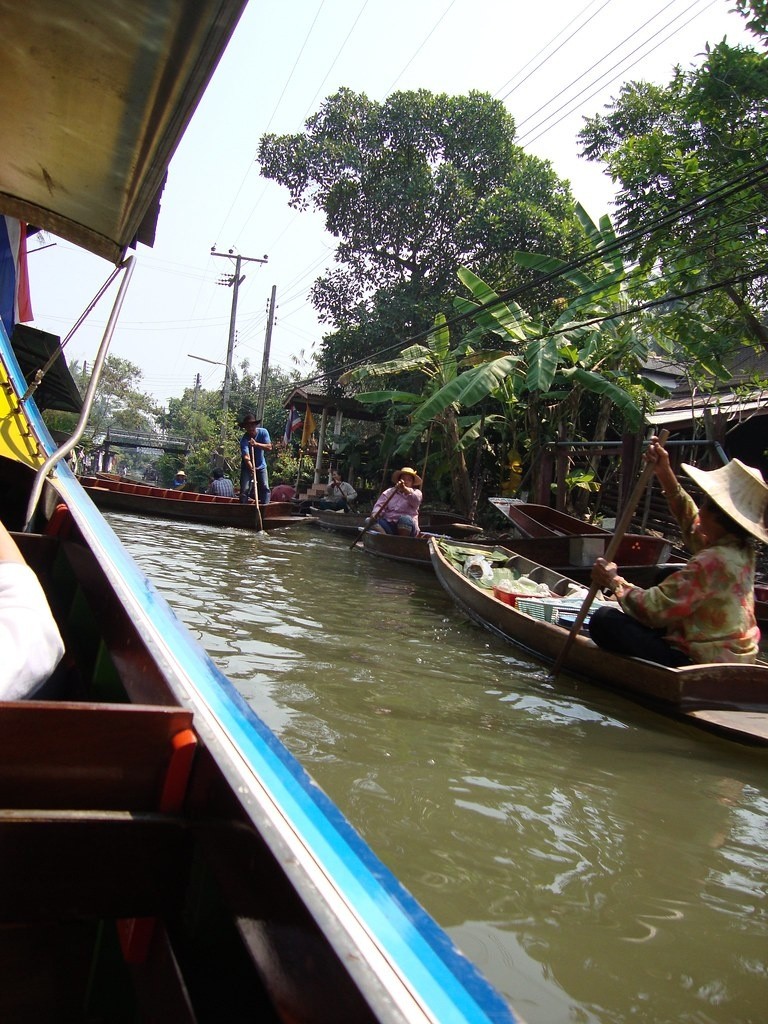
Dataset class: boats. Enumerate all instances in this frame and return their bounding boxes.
[353,527,675,596]
[428,536,768,749]
[306,506,484,535]
[75,472,320,531]
[488,497,768,637]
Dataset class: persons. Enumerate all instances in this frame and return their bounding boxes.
[588,441,768,667]
[364,466,423,538]
[121,465,127,477]
[209,468,235,497]
[205,477,215,494]
[238,413,273,504]
[143,470,148,475]
[173,470,187,489]
[319,470,358,514]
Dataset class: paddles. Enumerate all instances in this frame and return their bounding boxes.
[250,444,263,534]
[349,489,398,551]
[203,472,215,483]
[548,427,671,679]
[331,477,358,514]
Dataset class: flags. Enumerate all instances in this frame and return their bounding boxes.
[0,215,33,337]
[281,403,303,446]
[300,404,317,447]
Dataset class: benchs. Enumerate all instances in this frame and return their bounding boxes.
[1,700,197,824]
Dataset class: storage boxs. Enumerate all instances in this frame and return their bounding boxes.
[493,585,563,611]
[515,597,606,625]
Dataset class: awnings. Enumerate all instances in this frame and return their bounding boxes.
[0,0,249,414]
[3,320,92,416]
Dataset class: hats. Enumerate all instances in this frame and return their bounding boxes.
[176,471,186,475]
[238,413,261,428]
[392,467,422,485]
[681,458,768,547]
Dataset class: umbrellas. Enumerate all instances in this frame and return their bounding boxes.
[271,485,296,502]
